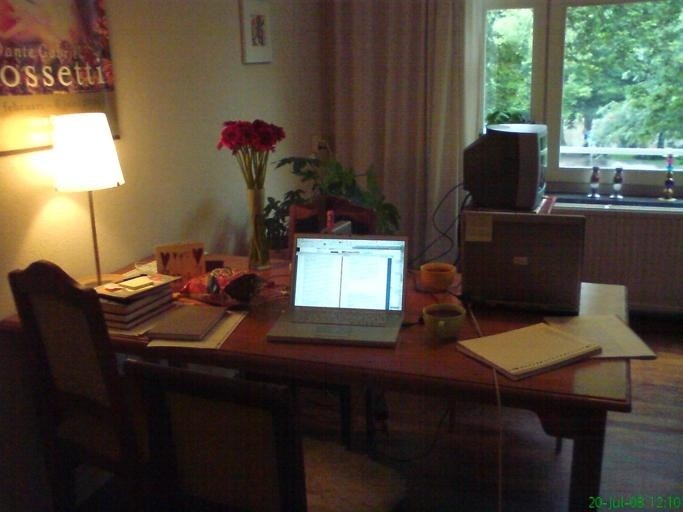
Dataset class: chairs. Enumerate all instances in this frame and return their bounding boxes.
[288,193,381,264]
[8,260,165,512]
[125,362,409,512]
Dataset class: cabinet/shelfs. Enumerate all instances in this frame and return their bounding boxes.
[459,210,585,317]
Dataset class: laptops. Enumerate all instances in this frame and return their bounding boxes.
[265,233,409,349]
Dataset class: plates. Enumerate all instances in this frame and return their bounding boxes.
[414,274,464,291]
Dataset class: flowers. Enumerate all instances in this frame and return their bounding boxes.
[216,119,285,190]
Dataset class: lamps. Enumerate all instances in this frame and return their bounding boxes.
[50,112,125,287]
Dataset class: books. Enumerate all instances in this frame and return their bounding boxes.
[148,305,226,340]
[93,271,181,331]
[456,322,603,381]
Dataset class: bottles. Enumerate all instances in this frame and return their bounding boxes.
[610,167,625,199]
[588,166,601,200]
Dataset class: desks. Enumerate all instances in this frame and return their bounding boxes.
[0,255,629,512]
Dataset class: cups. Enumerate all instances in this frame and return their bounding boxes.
[423,305,466,341]
[419,261,457,289]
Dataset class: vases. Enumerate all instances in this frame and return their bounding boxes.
[247,187,272,270]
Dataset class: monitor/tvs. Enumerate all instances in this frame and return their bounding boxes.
[462,123,548,211]
